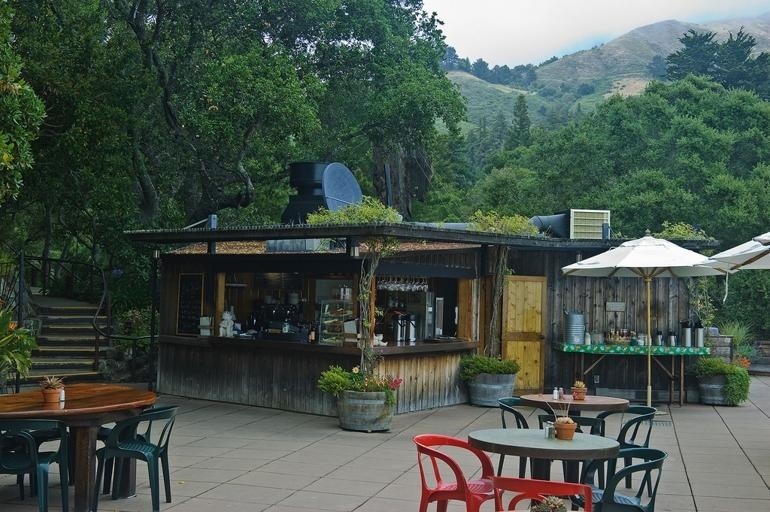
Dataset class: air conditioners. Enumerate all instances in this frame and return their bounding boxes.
[554,208,611,240]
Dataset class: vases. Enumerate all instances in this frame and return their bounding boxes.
[336,391,396,431]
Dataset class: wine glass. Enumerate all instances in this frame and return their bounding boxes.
[377,277,430,293]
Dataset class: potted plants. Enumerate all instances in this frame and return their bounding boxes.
[455,353,520,408]
[571,380,587,400]
[694,356,750,406]
[40,374,65,402]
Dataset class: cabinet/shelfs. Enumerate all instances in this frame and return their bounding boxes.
[320,299,355,348]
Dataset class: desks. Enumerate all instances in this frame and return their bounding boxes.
[0,386,156,512]
[519,394,629,482]
[468,427,620,511]
[554,343,710,406]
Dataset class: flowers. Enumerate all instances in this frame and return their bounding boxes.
[317,363,402,425]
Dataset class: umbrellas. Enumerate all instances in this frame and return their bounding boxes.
[692,229,769,273]
[556,234,721,414]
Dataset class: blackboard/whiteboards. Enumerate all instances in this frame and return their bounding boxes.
[176,273,204,336]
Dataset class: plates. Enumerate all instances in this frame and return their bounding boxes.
[327,311,353,317]
[288,292,299,305]
[264,295,272,305]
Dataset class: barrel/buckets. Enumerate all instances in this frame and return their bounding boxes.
[564,312,586,345]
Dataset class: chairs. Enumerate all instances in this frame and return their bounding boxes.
[491,475,592,511]
[412,434,503,512]
[539,414,605,490]
[581,448,667,512]
[89,406,179,512]
[590,406,656,497]
[1,418,70,512]
[497,397,532,478]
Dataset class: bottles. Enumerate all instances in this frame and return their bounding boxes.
[585,332,591,346]
[308,328,315,343]
[558,388,564,401]
[608,328,632,340]
[60,403,66,410]
[553,387,559,400]
[59,387,66,401]
[282,318,290,333]
[545,421,556,439]
